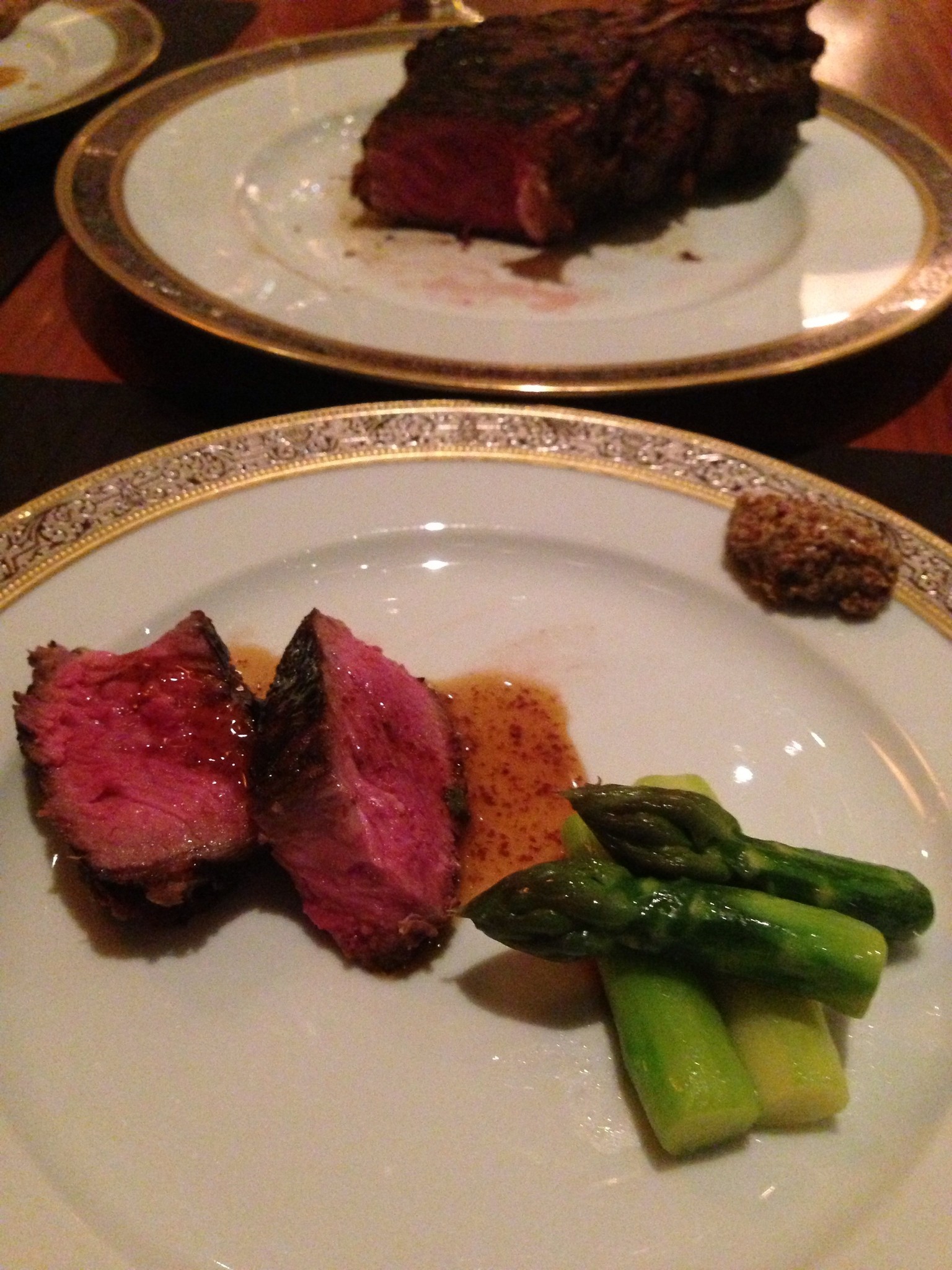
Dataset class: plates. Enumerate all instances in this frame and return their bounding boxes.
[58,12,950,393]
[3,0,162,124]
[0,396,952,1263]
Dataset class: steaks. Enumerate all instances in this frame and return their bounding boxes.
[350,0,826,248]
[12,609,261,927]
[261,608,463,980]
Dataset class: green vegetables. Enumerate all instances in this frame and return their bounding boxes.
[455,774,937,1154]
